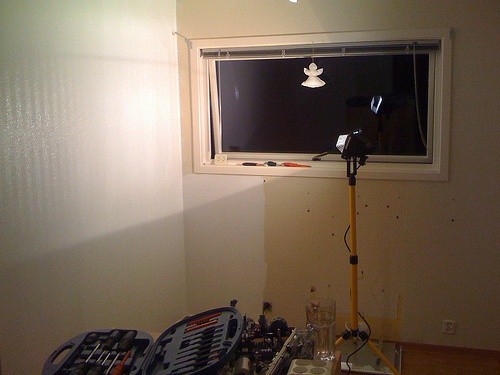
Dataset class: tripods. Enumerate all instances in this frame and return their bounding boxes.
[324,142,401,374]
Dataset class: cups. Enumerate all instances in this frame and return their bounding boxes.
[305,297,335,361]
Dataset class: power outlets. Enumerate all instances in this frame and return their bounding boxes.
[261,301,272,312]
[442,320,457,335]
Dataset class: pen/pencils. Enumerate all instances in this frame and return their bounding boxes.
[280,162,311,167]
[263,161,282,166]
[243,162,264,166]
